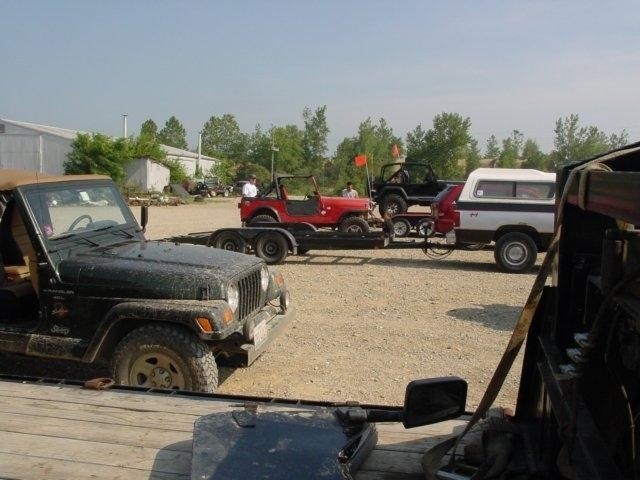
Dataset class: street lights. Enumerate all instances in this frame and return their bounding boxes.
[120,111,129,139]
[198,131,203,177]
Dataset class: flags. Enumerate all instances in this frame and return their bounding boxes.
[354,155,368,167]
[392,143,399,160]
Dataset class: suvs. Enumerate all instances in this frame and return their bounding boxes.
[449,164,558,267]
[183,173,264,198]
[370,160,468,217]
[0,168,287,399]
[238,173,375,237]
[432,182,552,237]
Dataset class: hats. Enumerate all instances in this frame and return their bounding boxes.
[249,174,256,179]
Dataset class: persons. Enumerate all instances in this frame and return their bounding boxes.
[241,173,259,200]
[341,182,358,198]
